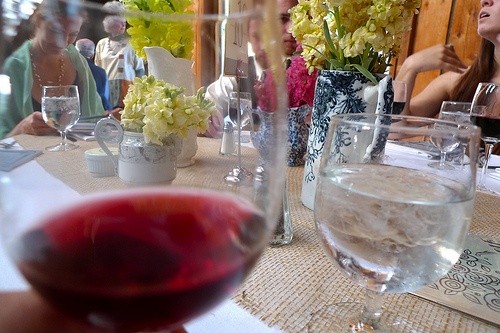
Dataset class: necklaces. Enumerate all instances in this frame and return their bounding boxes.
[108,36,123,55]
[31,50,64,92]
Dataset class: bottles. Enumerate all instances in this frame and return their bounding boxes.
[219,121,237,157]
[251,162,293,248]
[222,166,254,204]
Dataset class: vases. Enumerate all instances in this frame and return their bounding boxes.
[300,72,394,210]
[247,106,309,166]
[96,115,178,184]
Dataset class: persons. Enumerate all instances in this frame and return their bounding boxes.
[94,1,145,108]
[1,289,190,333]
[205,13,274,137]
[0,2,123,139]
[276,0,310,70]
[388,0,500,155]
[75,39,112,112]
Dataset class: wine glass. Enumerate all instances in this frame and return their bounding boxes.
[228,92,252,143]
[41,85,80,152]
[427,101,473,173]
[305,114,480,332]
[469,81,500,191]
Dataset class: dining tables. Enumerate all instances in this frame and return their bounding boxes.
[0,110,500,333]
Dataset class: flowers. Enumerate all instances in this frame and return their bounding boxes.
[253,53,313,107]
[123,0,197,59]
[121,77,213,133]
[285,0,425,71]
[139,47,197,167]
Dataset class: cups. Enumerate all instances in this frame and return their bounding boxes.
[85,146,118,177]
[392,81,407,114]
[0,0,289,332]
